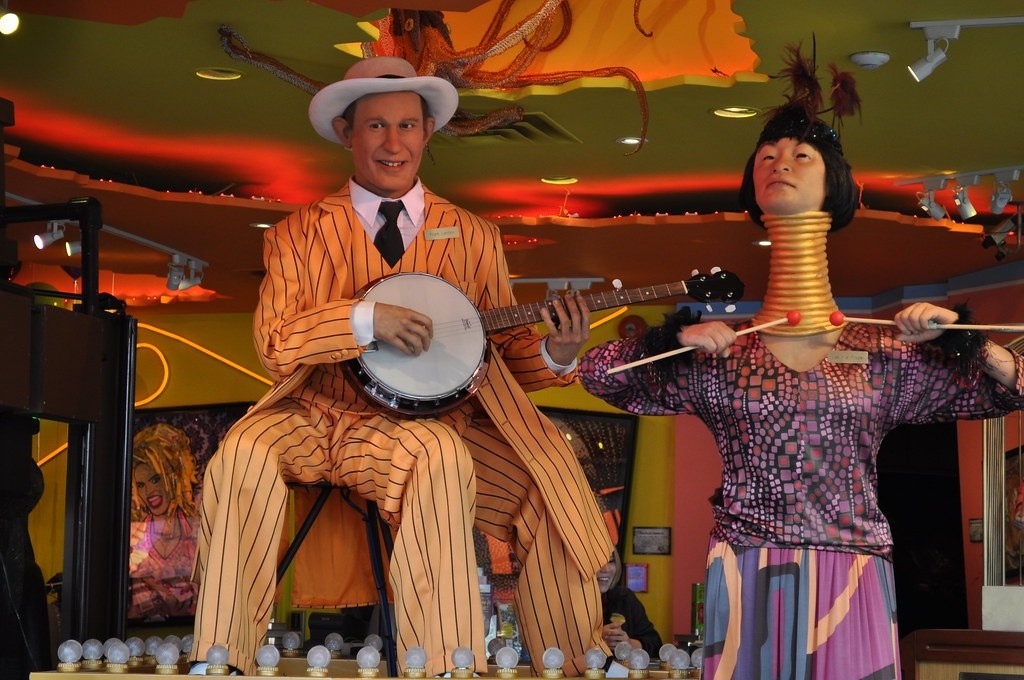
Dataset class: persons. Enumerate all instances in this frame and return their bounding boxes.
[578,112,1024,680]
[596,547,663,673]
[187,56,614,679]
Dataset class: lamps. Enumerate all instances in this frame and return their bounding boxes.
[65,227,82,257]
[166,255,204,290]
[915,178,948,221]
[33,220,65,249]
[952,175,979,221]
[990,169,1019,215]
[982,216,1019,262]
[907,25,963,82]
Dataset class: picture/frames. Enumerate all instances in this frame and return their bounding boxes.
[127,402,257,629]
[472,405,638,603]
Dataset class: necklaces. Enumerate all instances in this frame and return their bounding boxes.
[750,210,850,338]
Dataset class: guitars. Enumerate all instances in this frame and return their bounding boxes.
[336,266,748,421]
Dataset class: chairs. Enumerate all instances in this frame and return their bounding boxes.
[274,481,400,678]
